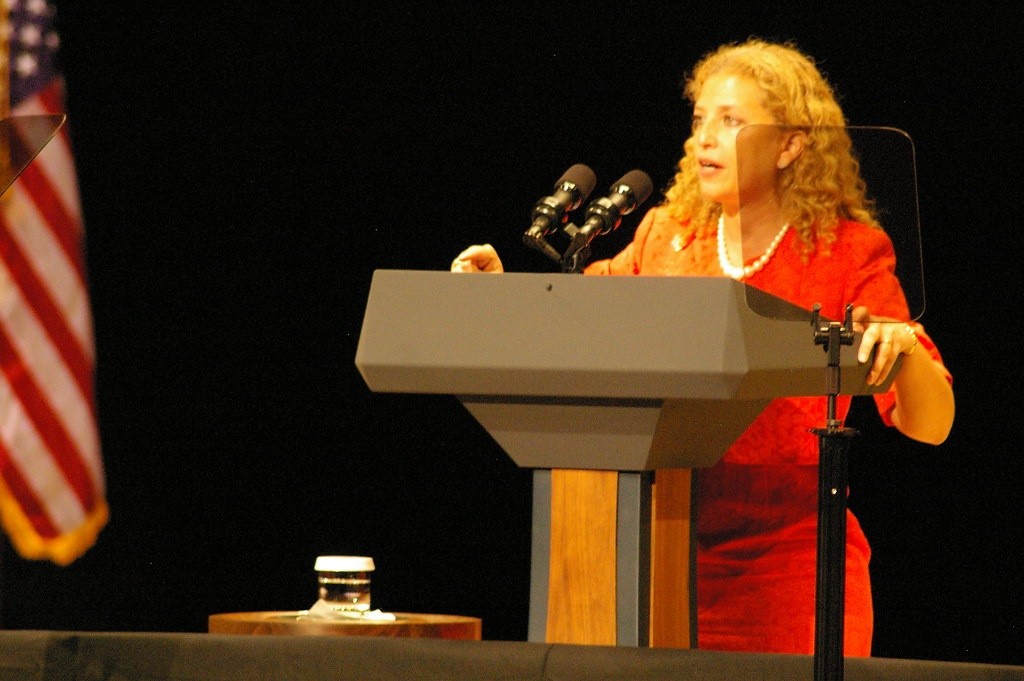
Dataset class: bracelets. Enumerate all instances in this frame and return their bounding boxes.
[904,324,919,356]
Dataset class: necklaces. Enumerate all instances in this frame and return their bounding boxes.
[716,213,793,281]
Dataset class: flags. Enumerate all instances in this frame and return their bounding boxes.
[0,1,109,564]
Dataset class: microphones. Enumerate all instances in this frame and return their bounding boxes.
[521,164,596,249]
[574,169,654,247]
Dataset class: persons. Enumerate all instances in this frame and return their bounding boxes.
[449,40,956,659]
